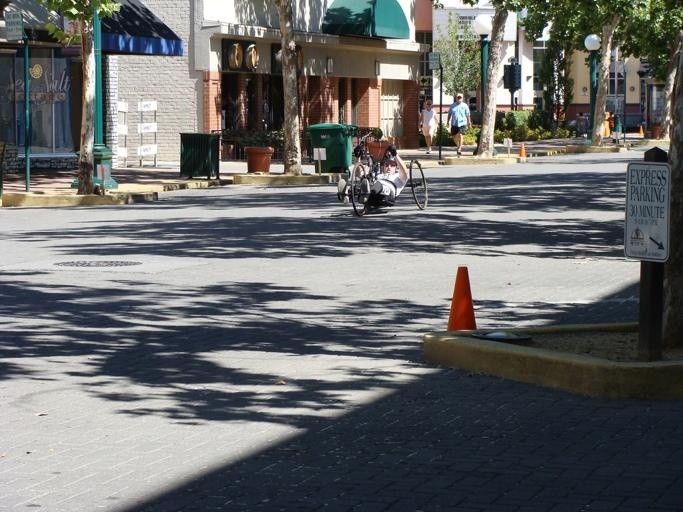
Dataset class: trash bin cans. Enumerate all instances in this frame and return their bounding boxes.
[309,123,358,173]
[180,133,221,180]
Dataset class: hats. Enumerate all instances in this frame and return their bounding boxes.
[383,156,398,166]
[456,93,463,98]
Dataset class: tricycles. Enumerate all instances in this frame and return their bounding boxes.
[337,144,428,217]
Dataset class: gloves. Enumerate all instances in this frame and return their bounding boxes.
[388,144,398,156]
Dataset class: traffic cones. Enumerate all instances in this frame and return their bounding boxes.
[639,125,643,135]
[448,267,476,330]
[520,144,526,157]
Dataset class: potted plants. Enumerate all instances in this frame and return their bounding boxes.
[367,127,390,162]
[221,126,285,172]
[653,116,661,139]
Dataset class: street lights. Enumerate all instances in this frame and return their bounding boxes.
[584,33,601,145]
[473,14,497,156]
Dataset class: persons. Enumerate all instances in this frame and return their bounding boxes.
[608,112,621,144]
[446,93,471,155]
[418,100,438,154]
[337,143,409,207]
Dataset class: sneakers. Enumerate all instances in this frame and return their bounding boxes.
[426,150,431,154]
[337,179,346,200]
[457,149,461,155]
[359,179,371,203]
